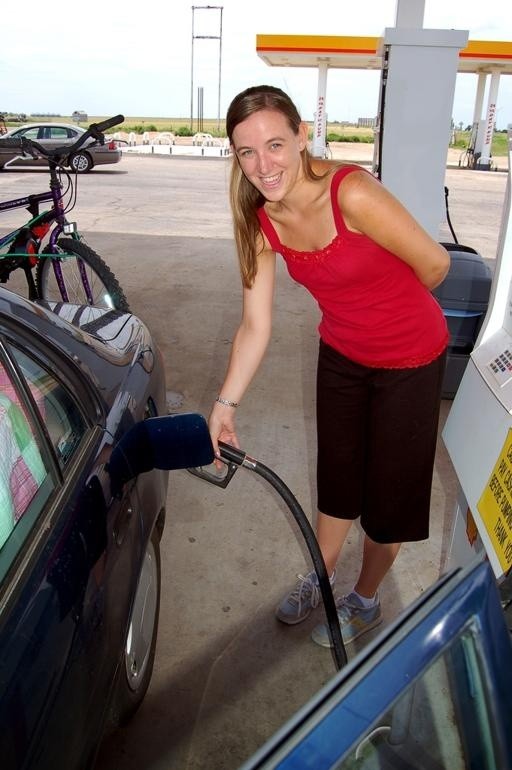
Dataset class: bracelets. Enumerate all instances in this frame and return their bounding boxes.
[216,397,242,409]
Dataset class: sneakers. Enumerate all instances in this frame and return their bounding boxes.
[275,567,337,626]
[311,592,384,647]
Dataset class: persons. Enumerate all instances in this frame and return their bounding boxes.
[199,81,452,646]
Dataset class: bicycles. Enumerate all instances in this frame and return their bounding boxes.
[0,115,133,314]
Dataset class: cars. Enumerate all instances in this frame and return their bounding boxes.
[0,286,214,770]
[0,122,122,173]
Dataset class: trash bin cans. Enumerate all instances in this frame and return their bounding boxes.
[432,251,492,399]
